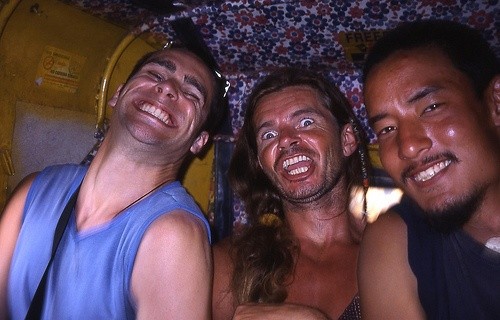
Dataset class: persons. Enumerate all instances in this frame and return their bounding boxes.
[0,45,222,320]
[357,20,500,319]
[210,66,375,320]
